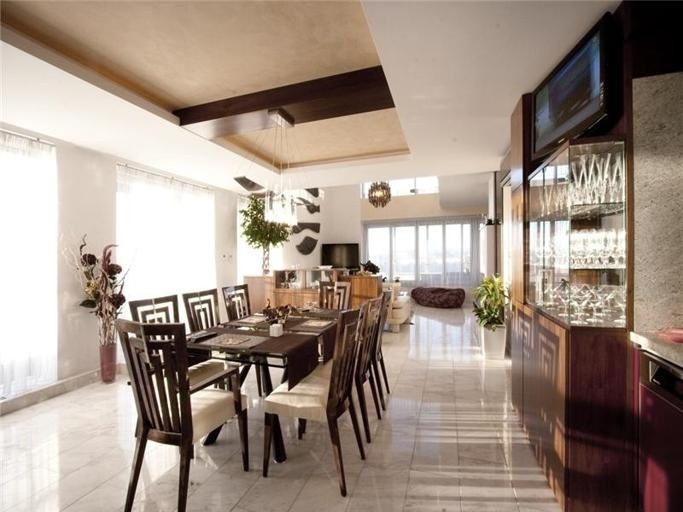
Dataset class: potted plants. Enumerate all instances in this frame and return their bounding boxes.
[240,192,293,272]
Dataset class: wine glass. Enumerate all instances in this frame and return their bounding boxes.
[534,227,625,270]
[536,284,626,326]
[538,151,624,217]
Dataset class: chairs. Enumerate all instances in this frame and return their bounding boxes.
[381,283,410,332]
[116,277,390,512]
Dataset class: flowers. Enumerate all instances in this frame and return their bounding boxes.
[58,233,129,344]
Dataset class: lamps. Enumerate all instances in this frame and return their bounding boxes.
[265,107,298,227]
[368,181,391,207]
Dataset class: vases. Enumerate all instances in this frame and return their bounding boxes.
[99,342,117,382]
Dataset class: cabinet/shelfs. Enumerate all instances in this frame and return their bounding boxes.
[507,93,634,512]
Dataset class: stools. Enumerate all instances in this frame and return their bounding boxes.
[411,286,465,309]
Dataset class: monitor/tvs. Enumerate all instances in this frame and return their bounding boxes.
[531,12,623,162]
